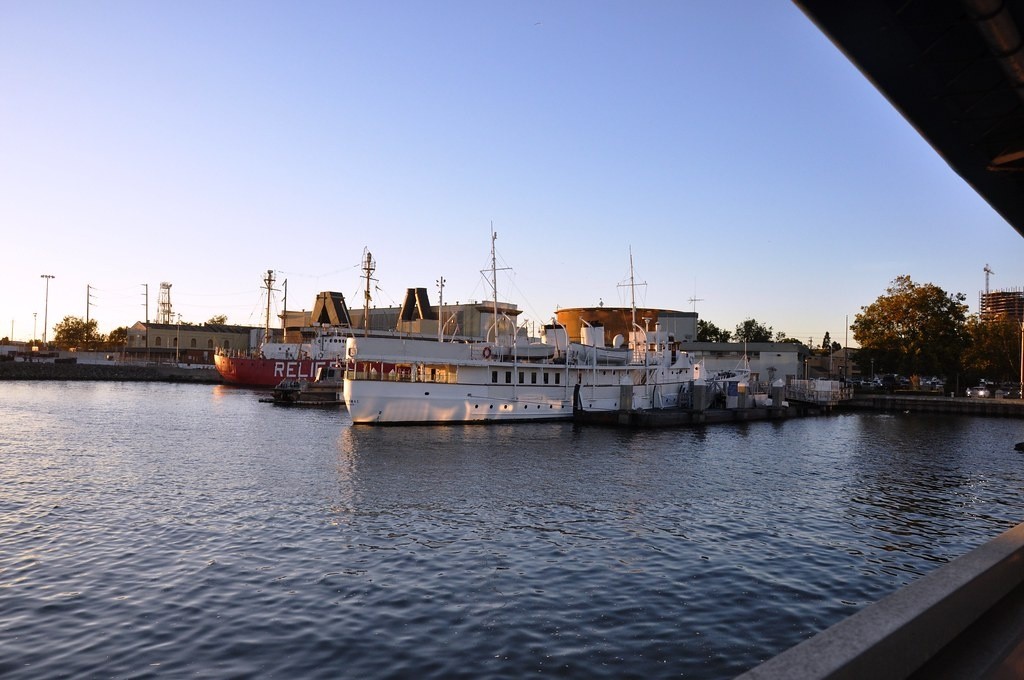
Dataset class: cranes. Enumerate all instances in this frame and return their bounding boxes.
[983,264,995,311]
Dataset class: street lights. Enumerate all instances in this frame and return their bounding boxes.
[40,273,56,345]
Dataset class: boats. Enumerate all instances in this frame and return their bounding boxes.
[213,225,857,424]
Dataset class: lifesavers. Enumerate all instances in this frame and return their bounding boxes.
[483,346,492,359]
[318,352,324,358]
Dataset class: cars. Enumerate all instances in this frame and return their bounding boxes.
[966,385,1021,399]
